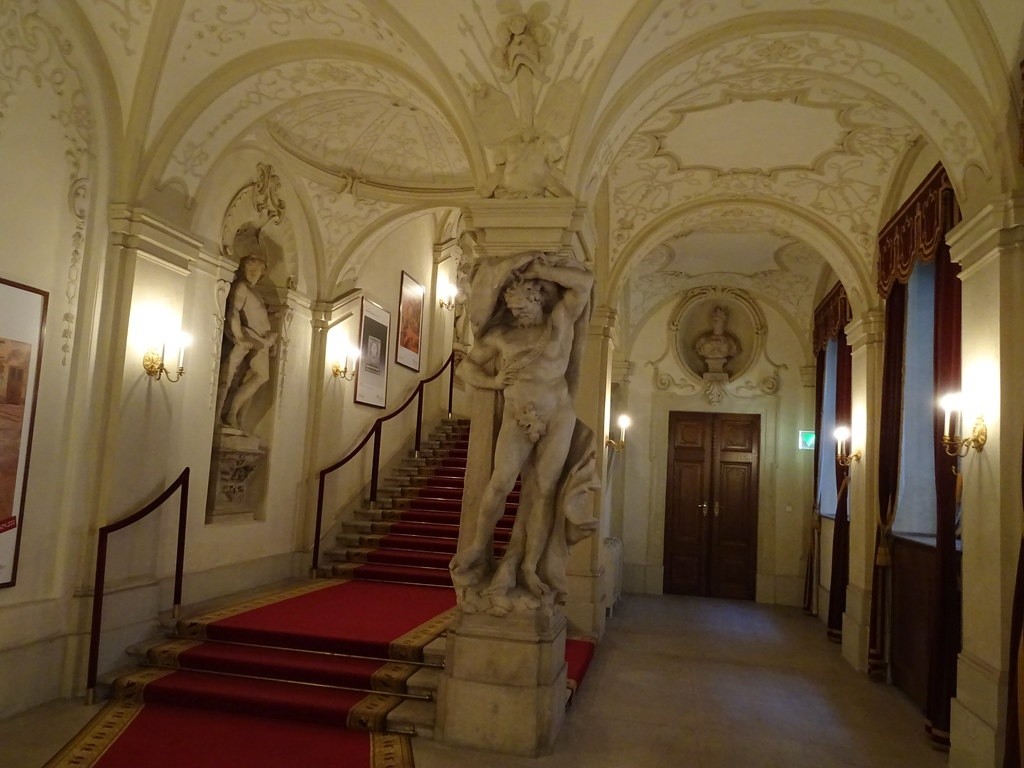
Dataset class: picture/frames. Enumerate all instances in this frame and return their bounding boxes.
[353,296,391,409]
[0,277,50,589]
[395,270,425,372]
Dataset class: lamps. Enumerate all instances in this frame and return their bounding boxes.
[143,329,194,381]
[606,415,631,452]
[439,285,458,311]
[939,392,988,457]
[834,426,861,467]
[333,345,361,381]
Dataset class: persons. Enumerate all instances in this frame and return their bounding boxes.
[448,257,595,597]
[693,306,741,357]
[224,252,278,438]
